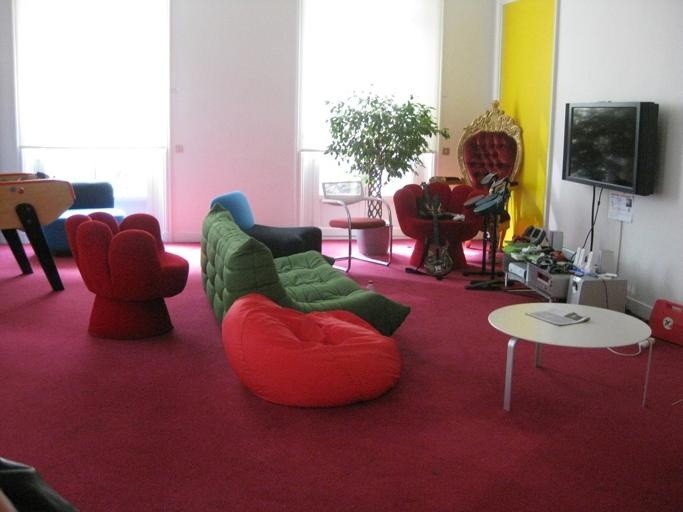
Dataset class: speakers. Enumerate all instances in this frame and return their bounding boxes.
[524,226,534,239]
[530,228,544,244]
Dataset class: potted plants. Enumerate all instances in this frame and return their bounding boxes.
[321,84,450,256]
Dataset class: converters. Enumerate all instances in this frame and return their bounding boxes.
[639,337,656,348]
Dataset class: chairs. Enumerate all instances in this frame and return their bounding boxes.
[456,99,524,254]
[210,190,335,265]
[220,292,401,408]
[63,212,189,340]
[393,182,487,271]
[321,181,393,273]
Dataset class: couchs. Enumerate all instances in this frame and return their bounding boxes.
[198,205,411,338]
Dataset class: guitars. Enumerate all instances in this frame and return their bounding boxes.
[416,182,453,275]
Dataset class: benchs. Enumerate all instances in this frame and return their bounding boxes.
[39,180,125,258]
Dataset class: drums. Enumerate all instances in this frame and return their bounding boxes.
[463,194,497,215]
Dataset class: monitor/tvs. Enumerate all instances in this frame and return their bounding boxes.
[561,101,658,196]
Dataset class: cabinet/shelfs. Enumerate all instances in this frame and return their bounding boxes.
[504,251,576,303]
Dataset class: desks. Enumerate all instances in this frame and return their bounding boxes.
[486,302,655,411]
